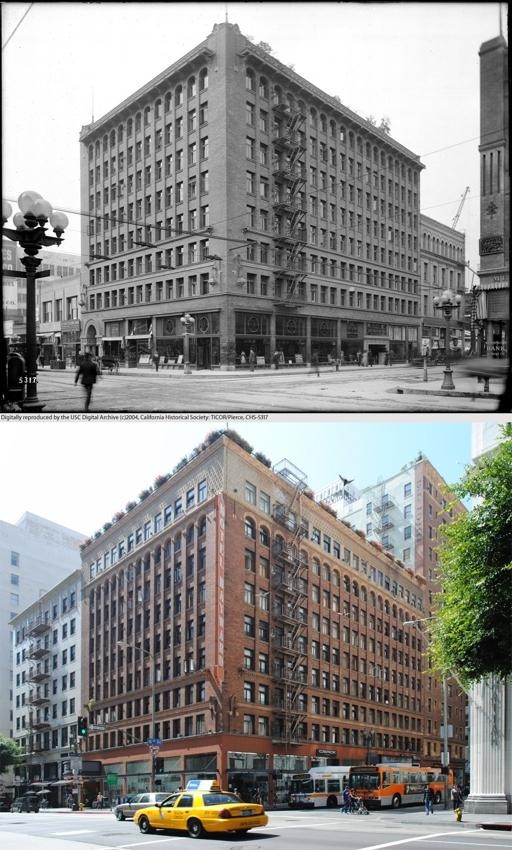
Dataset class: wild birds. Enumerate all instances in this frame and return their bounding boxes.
[339,475,354,486]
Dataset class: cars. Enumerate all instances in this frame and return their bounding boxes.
[113,779,269,838]
[0,795,40,813]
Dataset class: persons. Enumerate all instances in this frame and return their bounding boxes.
[423,782,434,815]
[451,783,463,810]
[233,787,241,797]
[249,349,255,372]
[341,786,360,815]
[74,351,101,411]
[356,349,373,367]
[389,348,394,367]
[96,791,107,809]
[152,351,160,372]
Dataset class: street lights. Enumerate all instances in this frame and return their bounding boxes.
[2,190,69,413]
[180,312,195,374]
[433,290,463,389]
[116,641,156,792]
[361,727,376,764]
[402,616,448,766]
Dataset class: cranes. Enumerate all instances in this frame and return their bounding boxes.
[451,186,469,229]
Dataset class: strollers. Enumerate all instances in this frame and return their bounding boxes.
[351,798,369,815]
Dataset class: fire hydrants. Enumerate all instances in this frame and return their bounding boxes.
[79,803,85,811]
[454,809,463,821]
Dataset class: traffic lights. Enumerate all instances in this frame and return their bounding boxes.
[77,716,89,737]
[157,758,164,774]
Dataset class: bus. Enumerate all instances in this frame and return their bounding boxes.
[289,763,453,811]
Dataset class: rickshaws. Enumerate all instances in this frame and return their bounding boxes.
[100,355,119,375]
